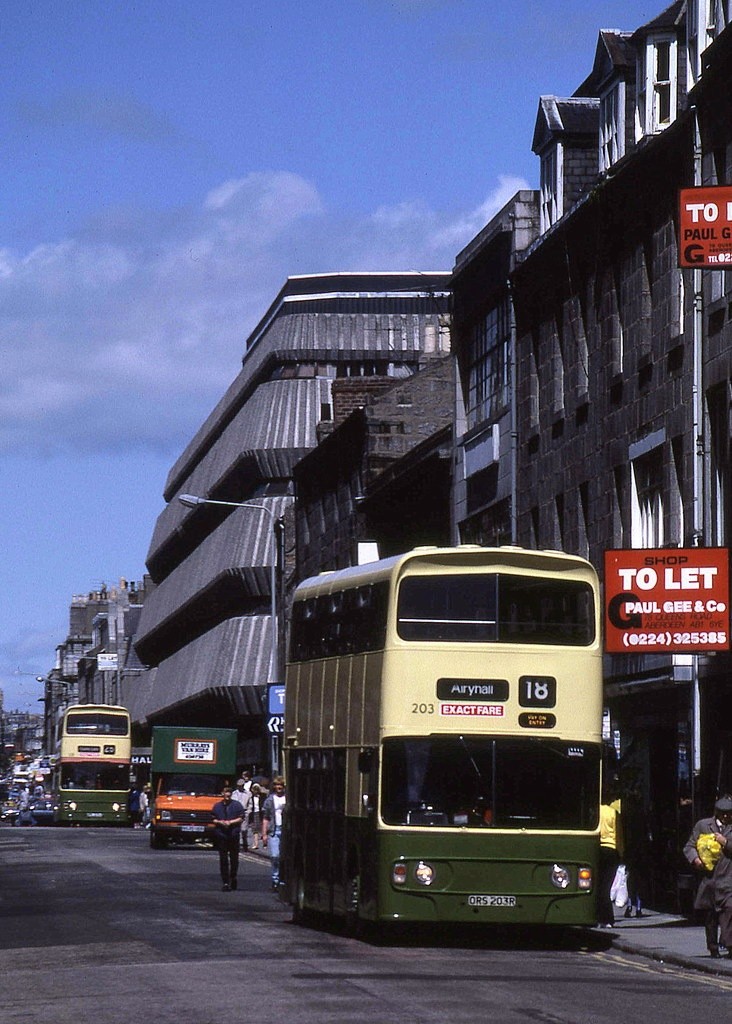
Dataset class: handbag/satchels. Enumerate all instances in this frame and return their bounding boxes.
[697,832,722,871]
[609,866,629,908]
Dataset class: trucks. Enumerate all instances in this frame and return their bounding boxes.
[146,725,243,854]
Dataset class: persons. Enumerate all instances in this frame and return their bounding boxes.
[16,786,37,827]
[683,798,732,959]
[231,779,253,852]
[211,787,245,890]
[597,795,622,929]
[248,783,268,850]
[139,786,150,826]
[252,768,269,786]
[262,780,287,887]
[131,785,141,829]
[242,771,252,791]
[623,789,651,916]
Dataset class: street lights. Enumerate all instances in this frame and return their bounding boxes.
[36,676,71,691]
[177,493,279,684]
[65,655,121,706]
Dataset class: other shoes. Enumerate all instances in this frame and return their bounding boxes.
[230,883,236,890]
[221,885,229,891]
[624,907,632,917]
[710,949,722,957]
[636,910,642,917]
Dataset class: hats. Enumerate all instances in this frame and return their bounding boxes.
[237,779,245,786]
[715,798,732,811]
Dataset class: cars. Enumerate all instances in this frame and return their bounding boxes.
[1,751,56,827]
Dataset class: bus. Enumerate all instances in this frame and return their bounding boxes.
[282,542,608,946]
[50,704,133,827]
